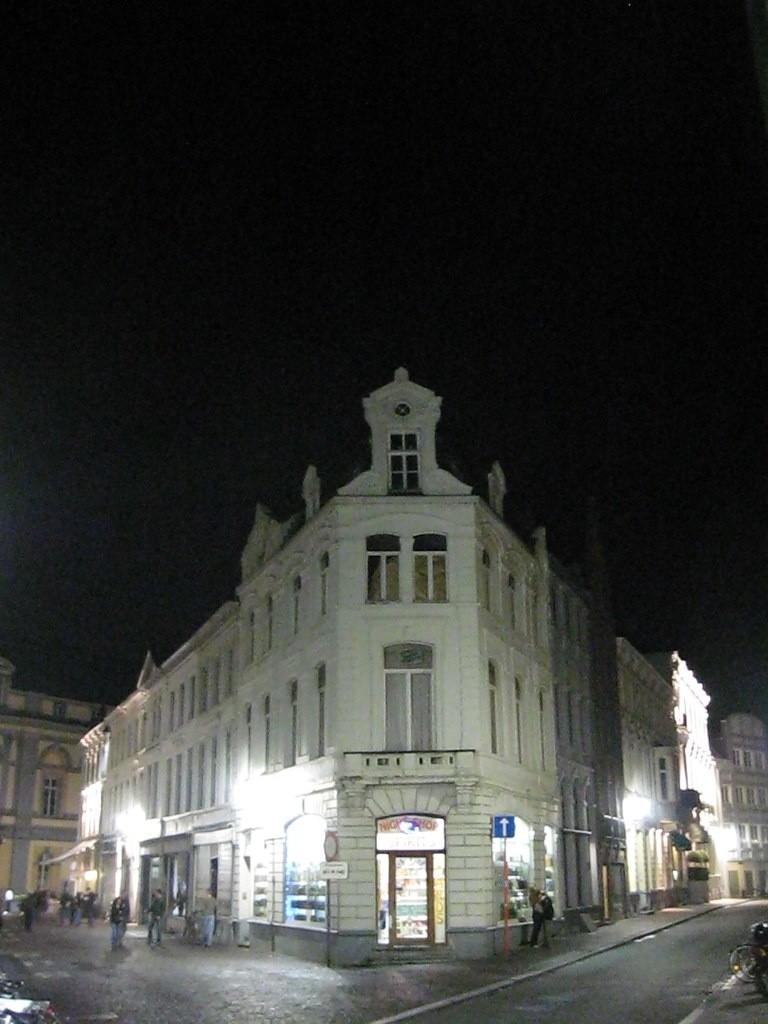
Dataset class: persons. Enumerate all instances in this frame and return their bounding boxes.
[141,887,166,945]
[530,888,554,951]
[21,883,50,932]
[108,889,131,949]
[55,884,73,921]
[69,892,82,926]
[201,888,219,947]
[82,888,96,925]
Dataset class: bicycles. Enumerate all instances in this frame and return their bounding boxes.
[729,945,761,984]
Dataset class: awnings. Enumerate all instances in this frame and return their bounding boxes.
[44,834,98,868]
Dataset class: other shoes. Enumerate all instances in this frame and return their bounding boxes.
[531,942,540,948]
